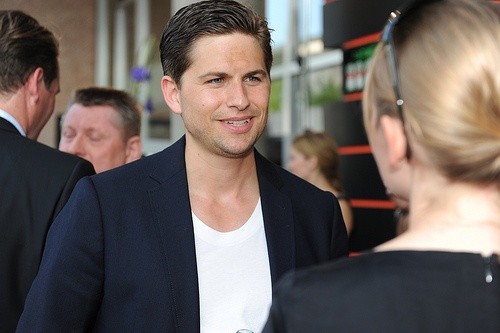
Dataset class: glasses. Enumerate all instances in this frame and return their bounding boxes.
[380,0,427,161]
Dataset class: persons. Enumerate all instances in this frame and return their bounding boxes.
[287,131,353,234]
[16,1,348,333]
[263,0,500,333]
[59,86,142,174]
[0,10,97,333]
